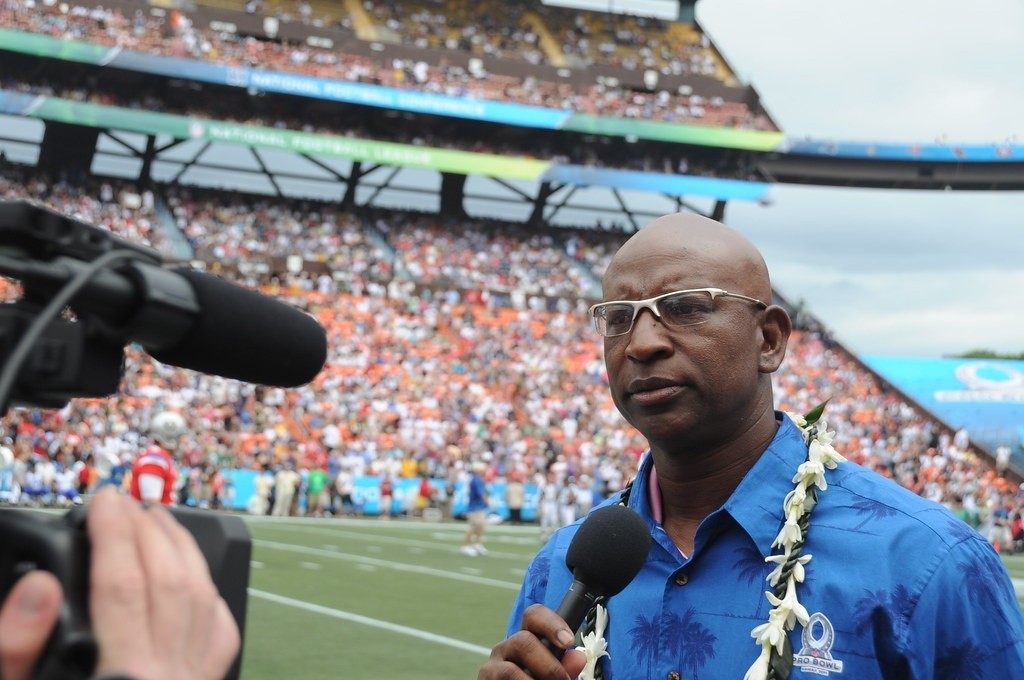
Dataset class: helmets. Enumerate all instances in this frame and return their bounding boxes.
[151,411,190,448]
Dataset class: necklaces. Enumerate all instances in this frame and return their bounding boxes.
[576,397,848,680]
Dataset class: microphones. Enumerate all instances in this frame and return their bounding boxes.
[53,257,327,389]
[523,505,652,680]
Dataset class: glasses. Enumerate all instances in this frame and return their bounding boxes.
[589,288,768,336]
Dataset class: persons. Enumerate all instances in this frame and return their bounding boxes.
[1,168,1023,552]
[1,1,782,181]
[1,487,241,680]
[477,213,1023,680]
